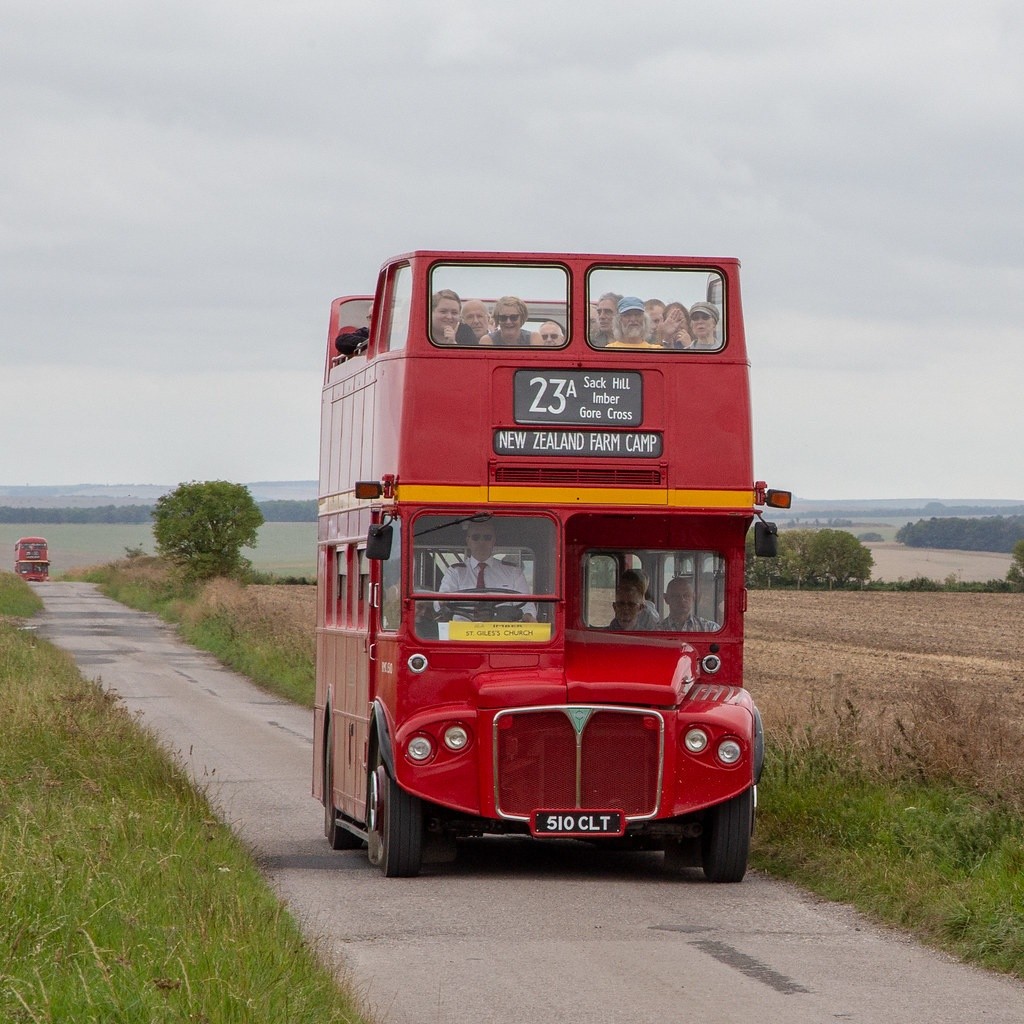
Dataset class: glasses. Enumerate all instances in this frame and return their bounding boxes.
[692,315,710,321]
[541,335,559,340]
[467,534,493,541]
[499,314,520,322]
[615,601,642,608]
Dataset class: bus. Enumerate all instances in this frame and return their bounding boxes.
[14,537,50,582]
[309,250,794,883]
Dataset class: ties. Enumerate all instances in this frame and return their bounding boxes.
[476,563,487,593]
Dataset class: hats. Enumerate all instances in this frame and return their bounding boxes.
[690,301,719,323]
[617,296,645,314]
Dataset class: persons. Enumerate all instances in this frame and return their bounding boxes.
[411,518,722,634]
[333,287,722,360]
